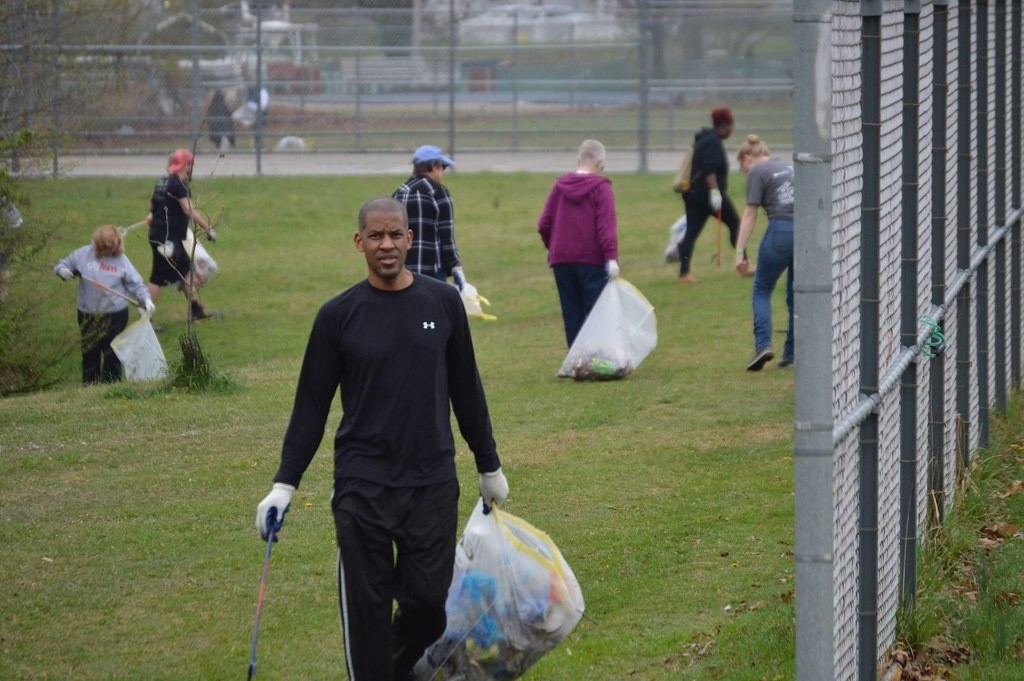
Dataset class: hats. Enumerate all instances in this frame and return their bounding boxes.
[413,144,454,170]
[165,149,194,173]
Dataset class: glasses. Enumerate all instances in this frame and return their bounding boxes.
[740,158,746,173]
[436,161,447,171]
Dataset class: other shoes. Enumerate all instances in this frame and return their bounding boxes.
[676,275,697,284]
[746,349,776,372]
[777,356,794,367]
[738,265,757,275]
[192,312,217,323]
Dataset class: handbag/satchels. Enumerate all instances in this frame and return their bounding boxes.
[672,141,697,192]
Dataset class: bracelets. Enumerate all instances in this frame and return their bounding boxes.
[710,188,720,192]
[736,247,745,254]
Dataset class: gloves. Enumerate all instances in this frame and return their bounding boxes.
[141,299,156,313]
[478,469,508,514]
[606,258,621,281]
[203,228,216,244]
[58,268,72,281]
[256,482,295,542]
[709,190,723,211]
[451,267,467,291]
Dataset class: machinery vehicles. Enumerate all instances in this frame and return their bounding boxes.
[133,14,325,97]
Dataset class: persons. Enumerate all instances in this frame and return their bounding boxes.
[143,149,217,333]
[54,225,158,388]
[675,108,759,283]
[254,195,511,681]
[206,92,236,163]
[536,137,620,347]
[733,130,794,368]
[245,82,269,133]
[392,144,465,293]
